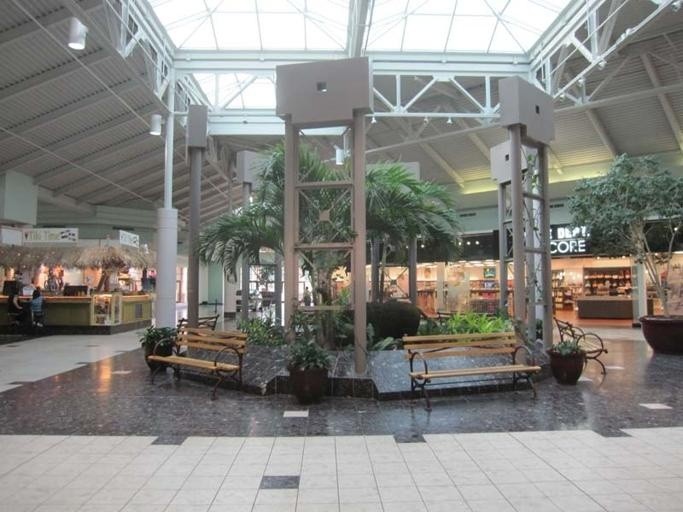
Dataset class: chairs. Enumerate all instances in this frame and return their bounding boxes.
[5,310,46,339]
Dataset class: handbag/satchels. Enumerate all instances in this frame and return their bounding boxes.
[584,271,633,296]
[555,287,564,309]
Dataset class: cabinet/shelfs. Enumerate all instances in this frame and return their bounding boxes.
[575,266,643,319]
[552,270,572,310]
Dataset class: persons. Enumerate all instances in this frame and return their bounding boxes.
[301,286,310,297]
[28,290,45,328]
[21,279,36,295]
[7,288,26,326]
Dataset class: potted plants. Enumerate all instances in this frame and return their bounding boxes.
[136,325,176,370]
[546,339,586,385]
[569,153,683,354]
[287,345,330,404]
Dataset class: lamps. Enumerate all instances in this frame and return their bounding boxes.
[149,114,165,135]
[336,149,349,165]
[67,17,89,50]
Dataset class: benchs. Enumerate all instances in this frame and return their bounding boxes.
[403,332,542,412]
[172,314,220,352]
[148,327,249,401]
[437,311,495,326]
[552,316,607,374]
